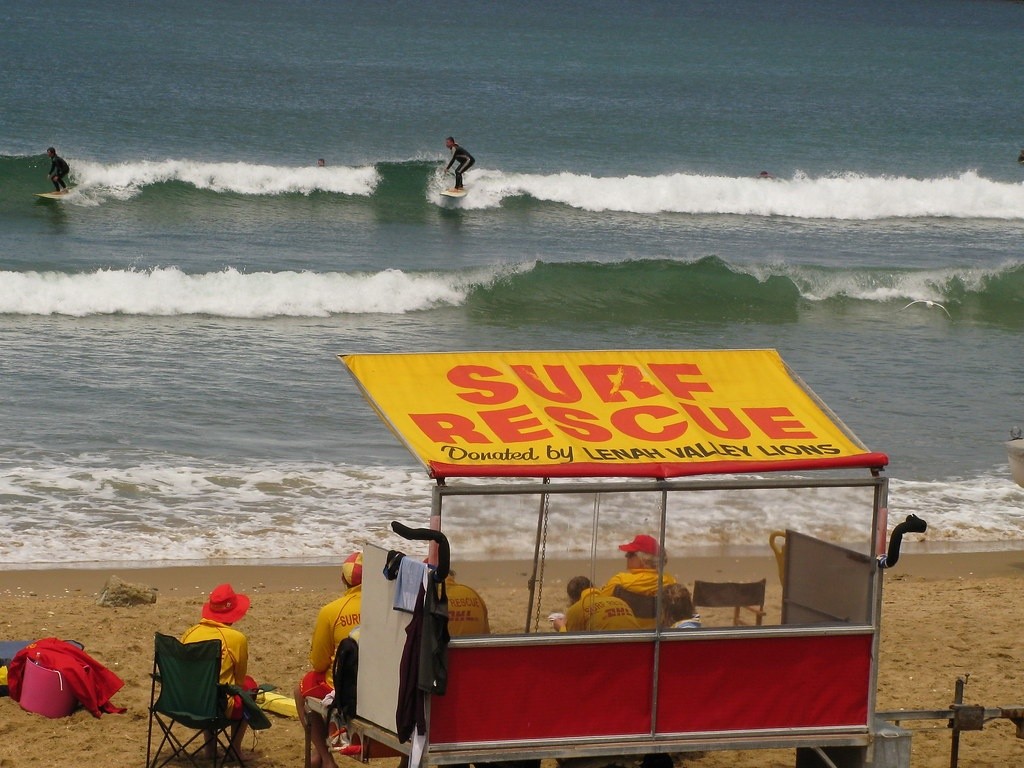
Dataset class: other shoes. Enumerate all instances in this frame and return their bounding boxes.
[640,752,674,768]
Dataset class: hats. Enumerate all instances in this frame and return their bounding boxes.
[202,583,250,623]
[342,551,362,586]
[619,535,659,555]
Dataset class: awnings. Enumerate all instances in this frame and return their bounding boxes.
[334,347,889,480]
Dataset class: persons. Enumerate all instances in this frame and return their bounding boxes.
[423,558,492,637]
[47,147,71,195]
[181,583,259,762]
[552,575,640,633]
[443,136,475,192]
[599,534,681,629]
[292,551,363,768]
[661,582,702,629]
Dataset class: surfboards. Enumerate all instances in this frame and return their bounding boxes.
[437,185,469,198]
[34,187,73,199]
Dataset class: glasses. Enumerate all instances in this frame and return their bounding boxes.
[627,552,637,559]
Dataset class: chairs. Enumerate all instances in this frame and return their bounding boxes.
[145,630,246,768]
[613,585,662,621]
[691,579,767,626]
